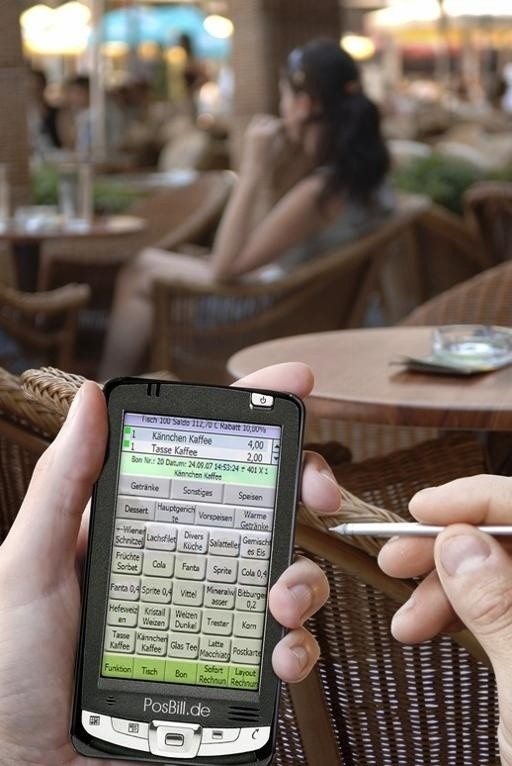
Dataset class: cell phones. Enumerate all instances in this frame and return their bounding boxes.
[68,377,307,766]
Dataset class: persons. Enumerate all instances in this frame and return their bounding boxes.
[381,32,512,169]
[143,31,206,101]
[1,361,511,765]
[32,69,228,170]
[95,39,392,379]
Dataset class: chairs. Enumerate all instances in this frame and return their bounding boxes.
[2,364,512,758]
[2,104,512,384]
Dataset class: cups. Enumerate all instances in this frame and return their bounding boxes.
[53,159,94,233]
